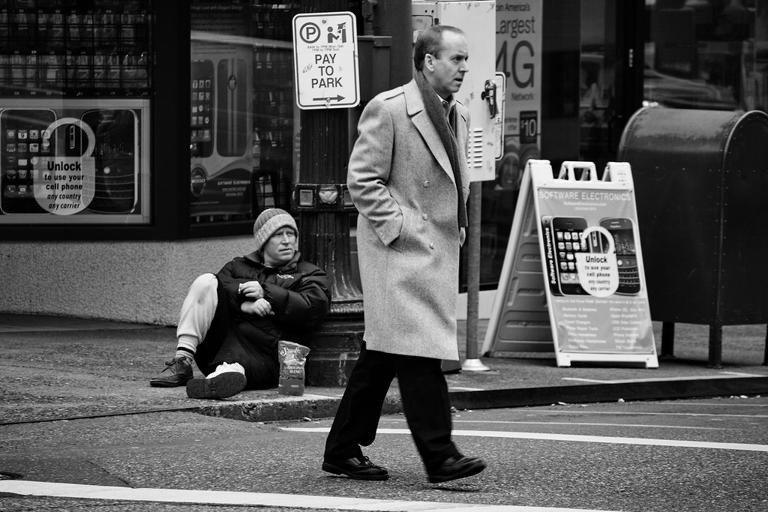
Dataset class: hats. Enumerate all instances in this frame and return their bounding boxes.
[253,208,300,249]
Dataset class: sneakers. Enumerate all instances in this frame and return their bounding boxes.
[186,371,246,399]
[150,357,193,387]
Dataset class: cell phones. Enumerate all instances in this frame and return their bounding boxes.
[1,107,58,214]
[217,58,247,156]
[191,58,214,160]
[551,217,585,296]
[542,215,559,298]
[79,108,140,214]
[600,216,640,296]
[65,123,81,158]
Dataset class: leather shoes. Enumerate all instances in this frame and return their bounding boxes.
[322,456,389,480]
[429,453,486,483]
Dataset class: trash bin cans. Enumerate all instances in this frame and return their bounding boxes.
[617,103,768,368]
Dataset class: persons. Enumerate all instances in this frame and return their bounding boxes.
[320,24,488,483]
[147,205,332,400]
